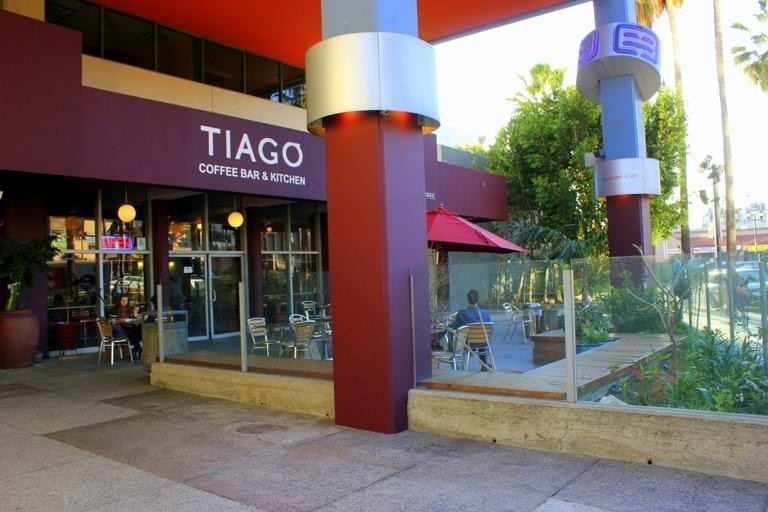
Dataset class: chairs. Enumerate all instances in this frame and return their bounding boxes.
[96,316,136,367]
[247,300,539,371]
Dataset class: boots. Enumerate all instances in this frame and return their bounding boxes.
[132,350,139,360]
[136,347,142,359]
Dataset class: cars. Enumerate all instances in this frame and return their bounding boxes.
[108,271,206,303]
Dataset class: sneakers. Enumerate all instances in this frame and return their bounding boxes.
[482,364,491,371]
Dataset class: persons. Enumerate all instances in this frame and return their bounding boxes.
[452,290,493,372]
[143,295,157,323]
[110,294,141,360]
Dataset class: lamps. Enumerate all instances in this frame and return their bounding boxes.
[117,182,137,223]
[228,193,244,228]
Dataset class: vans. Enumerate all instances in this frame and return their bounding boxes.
[706,266,768,313]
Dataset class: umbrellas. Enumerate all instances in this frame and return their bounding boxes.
[425,200,528,351]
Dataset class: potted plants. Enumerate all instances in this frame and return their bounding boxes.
[52,288,81,348]
[573,306,615,354]
[0,236,62,369]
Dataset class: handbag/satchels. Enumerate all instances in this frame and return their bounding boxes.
[110,321,125,337]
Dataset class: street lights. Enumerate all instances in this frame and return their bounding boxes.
[746,209,764,261]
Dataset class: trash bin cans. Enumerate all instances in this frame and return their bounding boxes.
[541,309,559,332]
[141,310,188,376]
